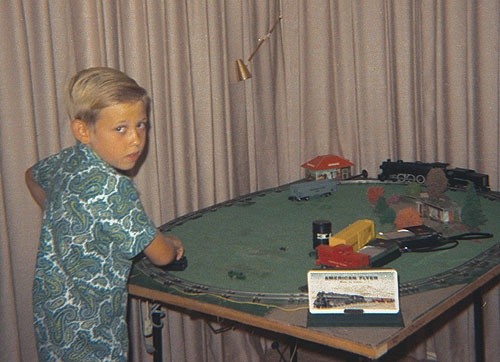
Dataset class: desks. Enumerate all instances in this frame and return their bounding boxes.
[125,174,500,362]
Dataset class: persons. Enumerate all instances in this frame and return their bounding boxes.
[25,66,184,362]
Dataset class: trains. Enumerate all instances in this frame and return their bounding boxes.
[314,292,395,309]
[378,158,490,195]
